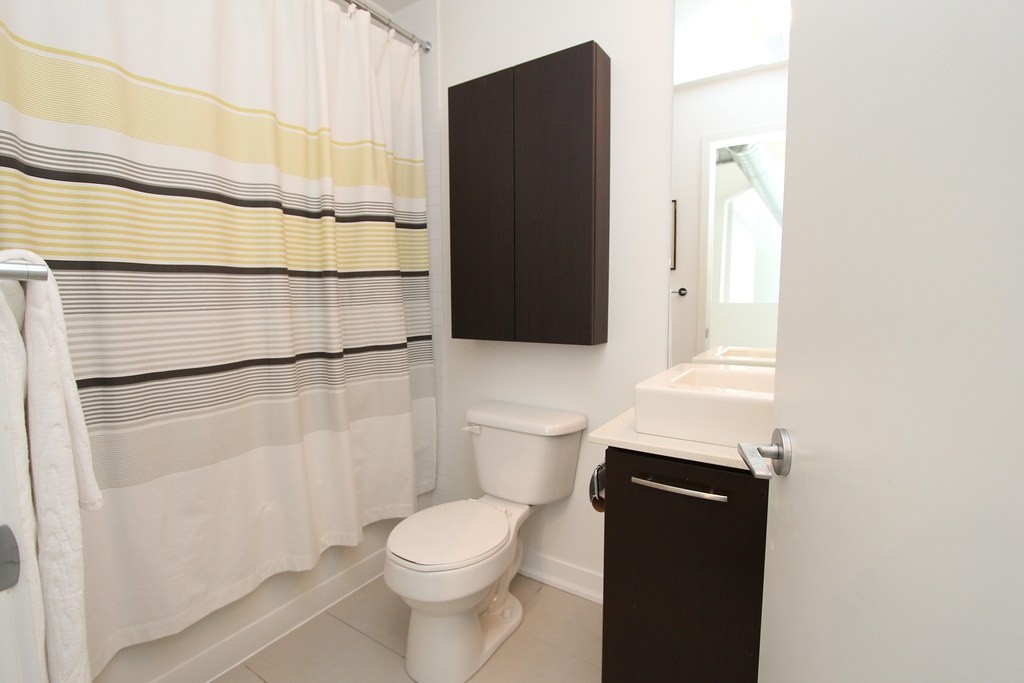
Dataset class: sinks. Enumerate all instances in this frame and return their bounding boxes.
[692,345,777,365]
[632,363,776,449]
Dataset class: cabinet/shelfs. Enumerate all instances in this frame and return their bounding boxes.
[601,446,770,683]
[449,40,611,345]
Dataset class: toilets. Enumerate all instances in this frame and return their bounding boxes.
[382,398,589,682]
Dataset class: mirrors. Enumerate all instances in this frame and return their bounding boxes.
[667,0,793,369]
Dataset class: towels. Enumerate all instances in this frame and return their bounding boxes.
[0,249,103,683]
